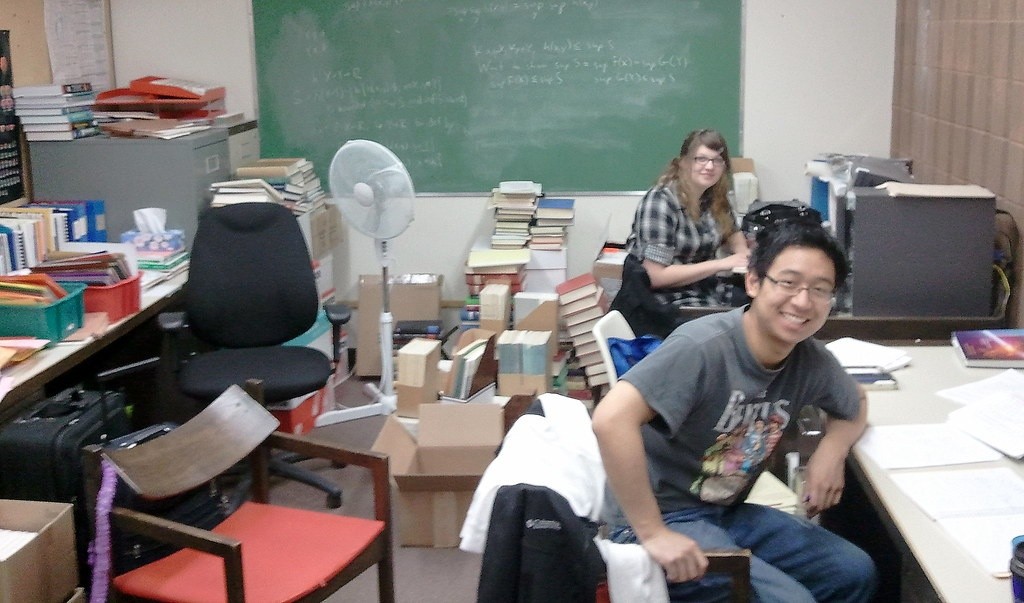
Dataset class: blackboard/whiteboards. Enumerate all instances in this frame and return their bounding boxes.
[254,0,745,199]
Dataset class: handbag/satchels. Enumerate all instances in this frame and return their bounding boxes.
[740,204,823,249]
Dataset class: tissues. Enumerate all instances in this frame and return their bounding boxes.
[120,207,185,252]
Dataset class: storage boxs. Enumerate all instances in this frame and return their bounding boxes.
[355,273,444,377]
[370,389,538,548]
[397,368,441,419]
[590,210,627,283]
[265,390,321,435]
[84,272,141,325]
[0,499,78,603]
[0,281,87,343]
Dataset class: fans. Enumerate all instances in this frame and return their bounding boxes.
[314,139,416,428]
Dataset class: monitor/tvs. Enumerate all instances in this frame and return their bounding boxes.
[825,178,858,313]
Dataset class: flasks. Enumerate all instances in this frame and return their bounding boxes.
[1009,542,1024,603]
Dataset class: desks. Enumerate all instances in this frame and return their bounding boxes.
[0,269,189,412]
[821,337,1024,603]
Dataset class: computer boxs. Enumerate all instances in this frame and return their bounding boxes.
[846,185,997,318]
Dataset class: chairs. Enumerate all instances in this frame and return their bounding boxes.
[82,378,396,603]
[460,389,760,603]
[157,202,352,509]
[592,309,638,389]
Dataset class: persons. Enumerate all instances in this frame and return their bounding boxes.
[627,130,751,315]
[592,218,879,603]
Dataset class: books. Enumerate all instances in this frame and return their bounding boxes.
[393,180,610,410]
[0,206,179,306]
[950,329,1024,368]
[852,373,896,392]
[12,83,99,141]
[207,158,326,215]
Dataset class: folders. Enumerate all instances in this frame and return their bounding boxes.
[24,201,107,244]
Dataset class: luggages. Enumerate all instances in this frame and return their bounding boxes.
[0,384,134,595]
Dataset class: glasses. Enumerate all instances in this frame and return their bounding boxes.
[692,157,727,167]
[759,271,838,305]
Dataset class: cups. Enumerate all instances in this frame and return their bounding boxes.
[1012,535,1024,551]
